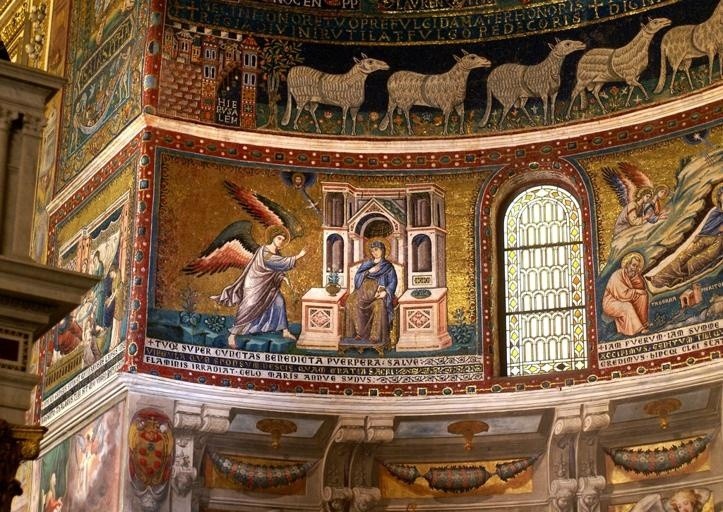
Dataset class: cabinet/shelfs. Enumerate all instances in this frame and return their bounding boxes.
[320,181,446,289]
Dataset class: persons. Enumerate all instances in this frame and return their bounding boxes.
[90,250,104,328]
[636,184,670,224]
[340,241,398,343]
[644,182,723,288]
[41,471,63,512]
[599,251,654,336]
[103,265,125,351]
[611,185,654,233]
[79,257,88,274]
[207,224,313,350]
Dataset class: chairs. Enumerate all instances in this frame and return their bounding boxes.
[340,260,403,356]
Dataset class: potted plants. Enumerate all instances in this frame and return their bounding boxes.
[326,272,340,296]
